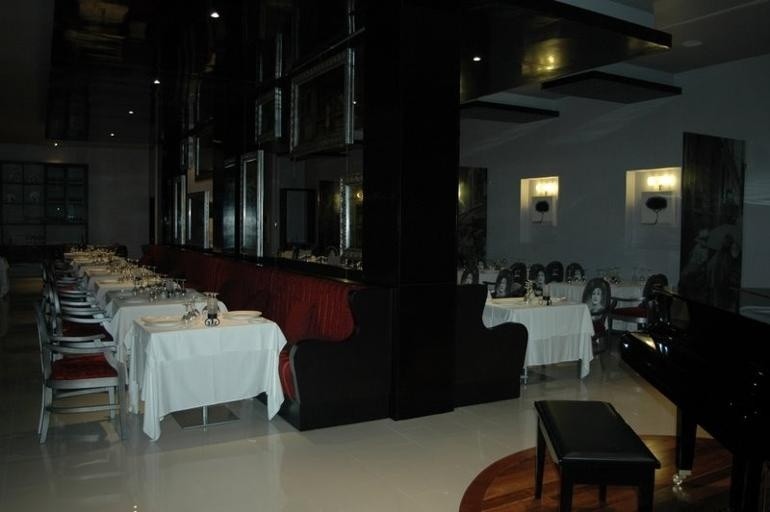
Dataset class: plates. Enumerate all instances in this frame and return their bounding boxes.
[141,315,180,327]
[222,310,262,321]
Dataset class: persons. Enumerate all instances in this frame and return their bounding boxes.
[460,258,611,315]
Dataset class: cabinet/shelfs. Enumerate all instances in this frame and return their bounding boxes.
[0,159,89,264]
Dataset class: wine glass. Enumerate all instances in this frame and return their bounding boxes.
[523,278,535,304]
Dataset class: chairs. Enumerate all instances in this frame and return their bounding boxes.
[28,245,130,443]
[456,255,673,386]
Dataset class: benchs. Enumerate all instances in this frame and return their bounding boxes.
[533,401,660,512]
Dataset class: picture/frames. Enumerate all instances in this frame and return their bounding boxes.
[253,0,356,162]
[172,136,210,249]
[221,149,265,268]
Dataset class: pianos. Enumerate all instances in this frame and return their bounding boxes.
[618,286,769,511]
